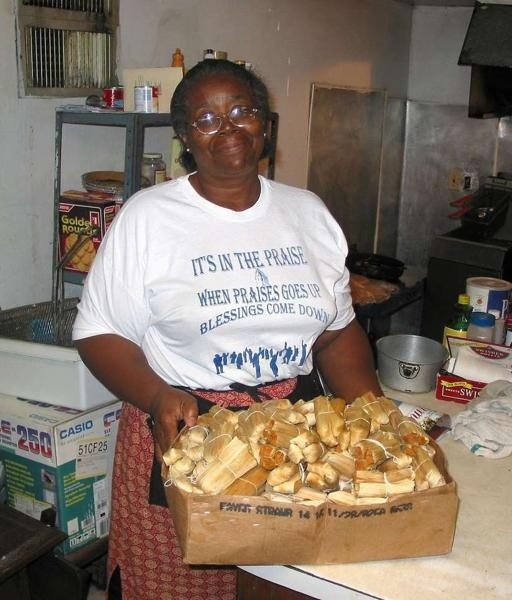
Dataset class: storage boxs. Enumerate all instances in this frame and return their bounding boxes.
[460,201,508,240]
[0,338,120,411]
[433,227,512,270]
[436,337,512,402]
[162,435,460,566]
[0,394,124,563]
[56,195,126,274]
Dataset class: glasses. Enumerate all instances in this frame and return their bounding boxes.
[184,103,266,136]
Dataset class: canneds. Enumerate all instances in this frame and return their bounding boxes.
[133,85,158,113]
[103,86,124,111]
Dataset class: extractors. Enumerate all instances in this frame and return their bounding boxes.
[458,4,509,118]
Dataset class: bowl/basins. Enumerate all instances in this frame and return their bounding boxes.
[375,334,449,392]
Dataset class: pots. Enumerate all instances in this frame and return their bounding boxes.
[347,252,404,279]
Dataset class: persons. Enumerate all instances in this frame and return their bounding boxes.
[71,58,388,600]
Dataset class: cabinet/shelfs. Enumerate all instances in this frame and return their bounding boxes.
[51,111,278,342]
[423,258,498,340]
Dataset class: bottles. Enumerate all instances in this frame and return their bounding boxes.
[171,48,184,76]
[142,154,166,184]
[443,294,473,349]
[205,49,214,60]
[489,309,506,345]
[466,312,495,344]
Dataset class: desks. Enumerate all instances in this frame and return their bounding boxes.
[44,535,108,600]
[0,502,68,600]
[353,268,427,360]
